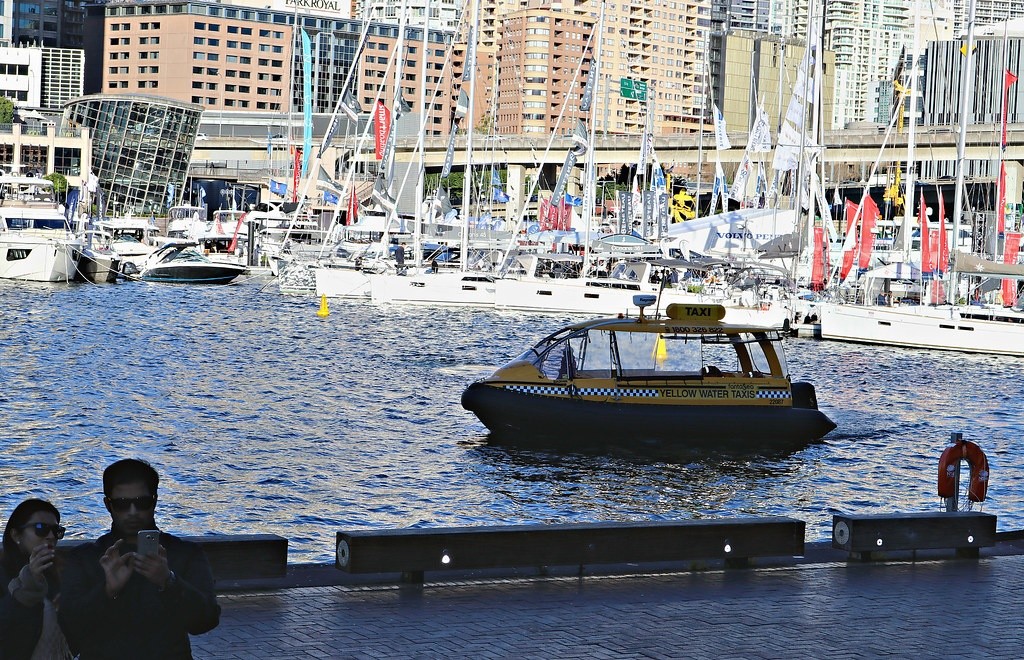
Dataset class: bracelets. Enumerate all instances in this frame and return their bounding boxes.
[158,571,175,593]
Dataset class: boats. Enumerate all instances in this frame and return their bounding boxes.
[166,203,289,265]
[86,218,161,270]
[461,295,838,444]
[133,241,246,286]
[0,173,84,281]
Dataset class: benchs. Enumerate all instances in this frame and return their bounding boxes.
[831,512,997,562]
[336,516,807,584]
[1,534,288,581]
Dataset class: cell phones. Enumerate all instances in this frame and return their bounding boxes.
[137,530,159,556]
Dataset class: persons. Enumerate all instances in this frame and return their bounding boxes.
[875,290,899,307]
[540,260,762,291]
[67,459,221,660]
[394,242,405,274]
[0,499,80,660]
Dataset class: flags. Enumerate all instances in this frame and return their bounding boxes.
[563,193,582,207]
[491,166,509,204]
[270,180,287,196]
[323,190,340,205]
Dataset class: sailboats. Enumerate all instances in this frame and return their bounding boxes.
[259,1,1024,356]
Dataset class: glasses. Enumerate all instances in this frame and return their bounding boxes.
[17,522,65,539]
[107,495,155,512]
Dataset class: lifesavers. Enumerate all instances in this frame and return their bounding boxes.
[937,440,990,502]
